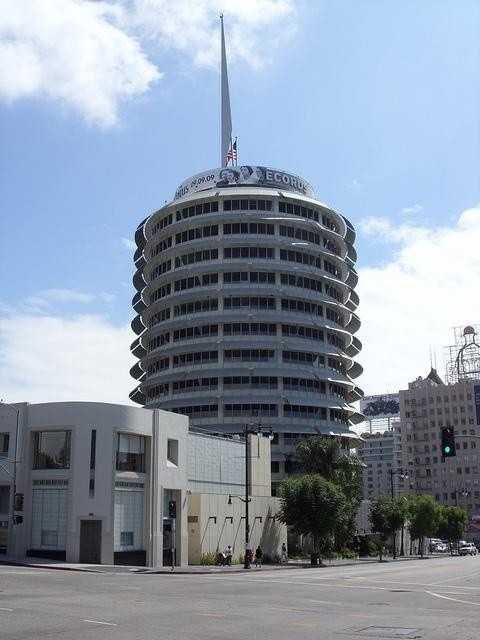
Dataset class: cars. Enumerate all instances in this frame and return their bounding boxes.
[429,538,476,556]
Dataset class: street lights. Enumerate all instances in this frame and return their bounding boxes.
[390,469,409,560]
[228,422,274,569]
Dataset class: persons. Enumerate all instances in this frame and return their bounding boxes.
[255,546,262,568]
[216,165,267,187]
[225,546,232,566]
[280,543,287,564]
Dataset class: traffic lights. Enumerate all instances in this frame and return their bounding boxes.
[442,426,456,456]
[168,501,176,518]
[15,496,24,510]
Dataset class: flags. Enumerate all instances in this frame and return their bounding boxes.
[225,142,237,165]
